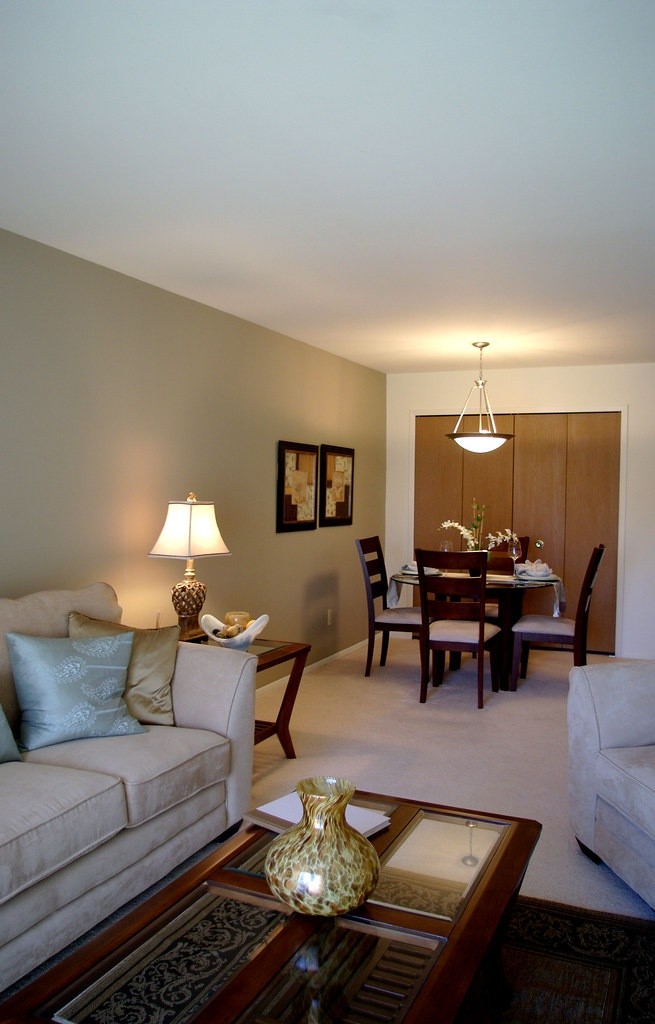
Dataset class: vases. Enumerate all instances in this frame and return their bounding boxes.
[467,550,487,576]
[265,775,383,918]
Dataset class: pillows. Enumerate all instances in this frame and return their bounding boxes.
[6,630,148,752]
[0,704,26,764]
[67,610,181,725]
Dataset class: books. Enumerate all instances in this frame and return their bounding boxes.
[244,791,391,838]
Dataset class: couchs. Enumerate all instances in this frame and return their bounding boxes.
[0,582,257,992]
[567,657,655,911]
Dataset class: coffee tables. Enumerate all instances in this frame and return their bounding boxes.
[0,781,539,1024]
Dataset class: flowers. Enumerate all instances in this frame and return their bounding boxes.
[438,497,517,555]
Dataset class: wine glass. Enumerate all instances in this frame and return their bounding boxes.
[439,540,453,577]
[508,541,522,580]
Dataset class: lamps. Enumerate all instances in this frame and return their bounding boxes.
[148,494,228,637]
[445,342,515,455]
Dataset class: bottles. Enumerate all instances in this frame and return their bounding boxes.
[264,775,381,916]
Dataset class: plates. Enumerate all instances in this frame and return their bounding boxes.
[407,564,427,572]
[525,571,549,577]
[518,574,557,581]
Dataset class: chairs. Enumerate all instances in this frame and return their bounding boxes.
[413,549,514,709]
[355,535,420,677]
[508,543,607,688]
[472,535,530,659]
[447,550,518,673]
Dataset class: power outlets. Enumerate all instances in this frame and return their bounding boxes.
[328,610,333,626]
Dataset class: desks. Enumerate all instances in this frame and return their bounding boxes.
[382,572,561,692]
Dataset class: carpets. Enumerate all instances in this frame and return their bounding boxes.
[0,826,655,1024]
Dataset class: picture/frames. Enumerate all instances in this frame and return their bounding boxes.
[275,441,319,533]
[319,444,355,527]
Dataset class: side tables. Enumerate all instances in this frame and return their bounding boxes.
[156,625,311,758]
